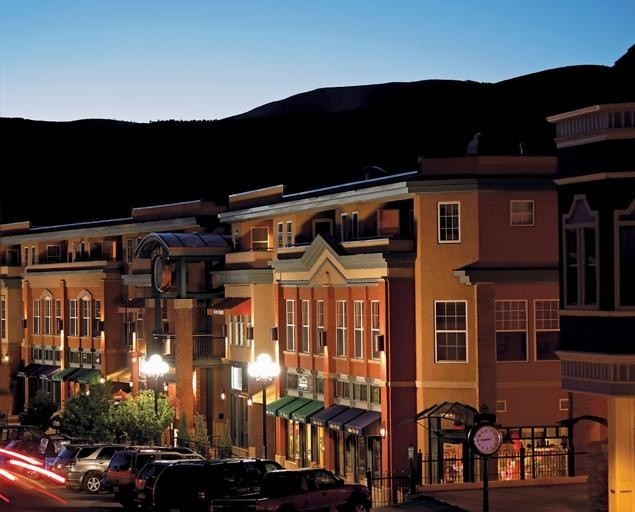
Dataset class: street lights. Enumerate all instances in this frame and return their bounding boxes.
[248,354,281,459]
[138,353,173,416]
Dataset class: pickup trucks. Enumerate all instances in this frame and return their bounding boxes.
[209,466,372,512]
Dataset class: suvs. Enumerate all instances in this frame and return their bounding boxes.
[131,458,203,511]
[150,457,286,511]
[100,449,207,509]
[0,422,131,494]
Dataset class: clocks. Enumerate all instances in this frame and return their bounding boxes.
[467,425,502,455]
